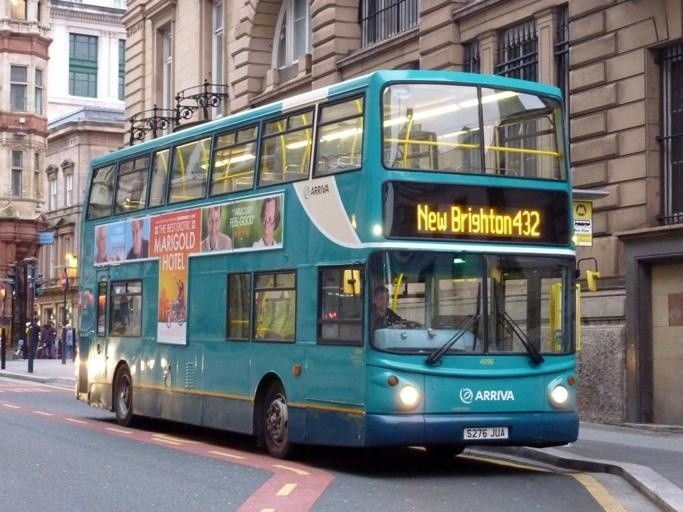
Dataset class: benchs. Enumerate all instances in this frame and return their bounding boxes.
[229,156,399,193]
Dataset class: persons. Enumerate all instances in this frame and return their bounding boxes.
[200,206,233,253]
[253,197,280,248]
[126,220,148,259]
[96,226,108,263]
[370,286,418,329]
[37,320,55,359]
[65,324,73,359]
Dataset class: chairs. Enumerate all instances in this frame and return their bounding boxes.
[229,300,295,340]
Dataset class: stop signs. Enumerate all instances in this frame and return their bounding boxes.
[61,273,67,290]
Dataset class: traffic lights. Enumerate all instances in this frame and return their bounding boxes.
[4,263,16,289]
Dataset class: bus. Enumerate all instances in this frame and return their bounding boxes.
[76,69,601,459]
